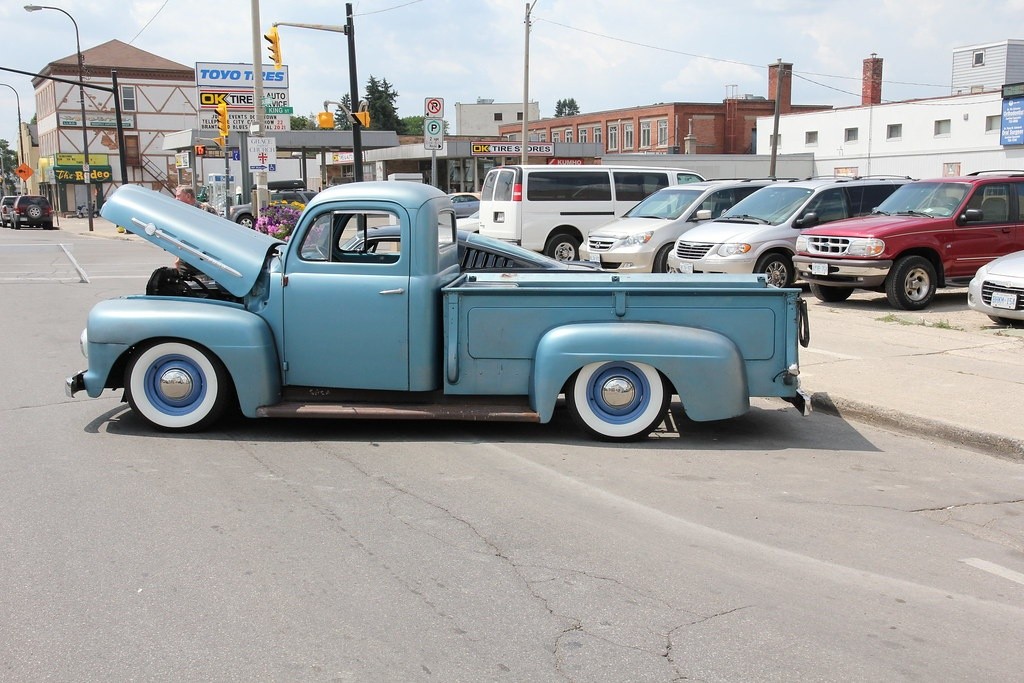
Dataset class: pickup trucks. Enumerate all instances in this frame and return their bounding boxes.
[61,182,810,440]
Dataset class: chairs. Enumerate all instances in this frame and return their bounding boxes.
[935,197,959,210]
[980,197,1006,221]
[713,191,732,217]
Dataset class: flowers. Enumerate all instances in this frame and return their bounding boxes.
[255,199,323,246]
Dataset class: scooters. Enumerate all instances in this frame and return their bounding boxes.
[77,205,99,219]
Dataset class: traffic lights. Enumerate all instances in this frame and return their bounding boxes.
[195,146,205,156]
[264,28,281,69]
[211,138,222,147]
[351,112,370,128]
[214,103,228,136]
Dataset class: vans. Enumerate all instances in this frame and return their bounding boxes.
[666,173,916,290]
[577,179,796,273]
[478,166,705,263]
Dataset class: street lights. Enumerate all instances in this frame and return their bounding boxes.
[24,5,92,232]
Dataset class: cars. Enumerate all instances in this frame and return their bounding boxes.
[967,250,1024,324]
[445,193,481,233]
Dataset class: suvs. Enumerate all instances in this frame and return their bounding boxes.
[790,170,1024,311]
[230,189,318,230]
[0,196,17,227]
[10,195,53,230]
